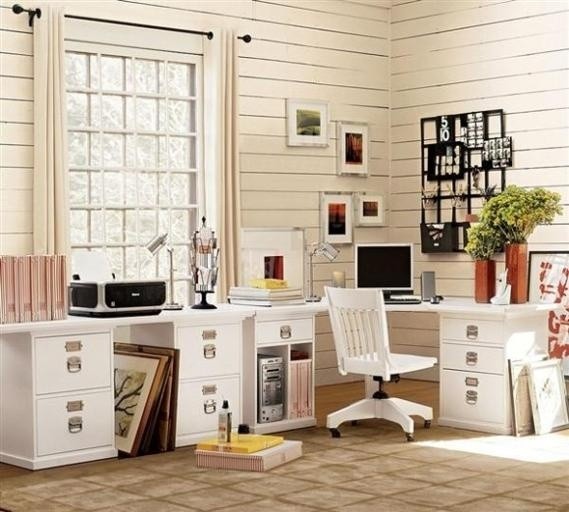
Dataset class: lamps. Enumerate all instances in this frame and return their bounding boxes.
[306,242,340,302]
[145,233,184,311]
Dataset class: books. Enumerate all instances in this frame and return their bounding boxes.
[227,287,305,307]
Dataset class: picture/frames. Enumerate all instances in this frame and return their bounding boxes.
[114,341,180,456]
[355,190,385,229]
[336,119,369,176]
[285,97,329,147]
[317,191,353,246]
[509,354,569,436]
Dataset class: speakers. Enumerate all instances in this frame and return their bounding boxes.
[421,272,435,300]
[332,270,345,288]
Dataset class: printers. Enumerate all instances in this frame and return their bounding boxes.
[68,252,166,316]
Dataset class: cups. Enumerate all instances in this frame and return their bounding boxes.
[330,270,346,289]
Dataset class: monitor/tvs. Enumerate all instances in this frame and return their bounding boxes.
[354,242,415,298]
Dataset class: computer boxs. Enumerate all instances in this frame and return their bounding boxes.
[257,353,285,423]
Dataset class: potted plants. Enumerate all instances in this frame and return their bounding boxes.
[480,185,563,302]
[463,225,495,303]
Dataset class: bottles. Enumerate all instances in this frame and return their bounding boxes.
[218,400,232,443]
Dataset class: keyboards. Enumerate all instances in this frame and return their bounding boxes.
[384,299,421,304]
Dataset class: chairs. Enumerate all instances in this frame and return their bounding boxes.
[322,284,437,441]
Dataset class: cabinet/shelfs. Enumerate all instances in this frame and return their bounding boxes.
[441,316,504,428]
[33,331,113,456]
[256,314,314,425]
[175,321,242,435]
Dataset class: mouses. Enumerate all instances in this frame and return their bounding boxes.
[430,296,440,303]
